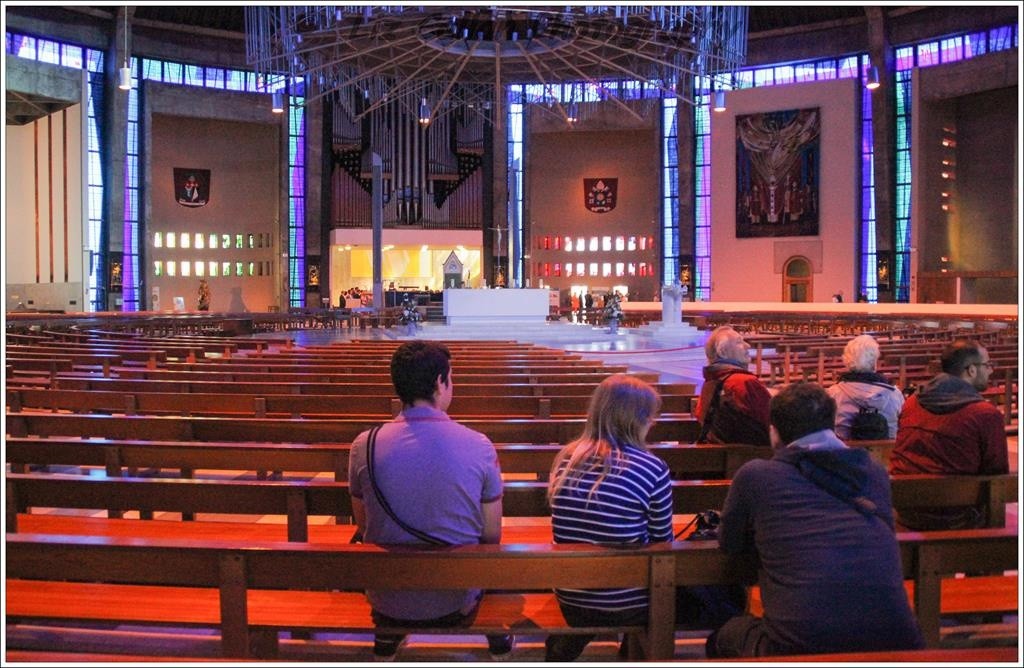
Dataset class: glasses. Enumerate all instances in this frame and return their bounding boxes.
[964,361,996,372]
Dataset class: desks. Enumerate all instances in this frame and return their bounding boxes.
[442,288,550,324]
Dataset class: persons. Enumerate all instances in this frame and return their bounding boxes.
[603,288,625,331]
[571,290,593,309]
[694,324,773,443]
[197,279,211,311]
[347,340,517,663]
[888,339,1010,530]
[543,373,674,662]
[339,286,364,309]
[823,335,906,439]
[703,381,922,658]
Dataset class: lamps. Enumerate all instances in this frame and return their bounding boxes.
[864,48,881,90]
[568,83,579,122]
[271,7,284,114]
[118,6,132,91]
[714,85,726,113]
[420,81,432,124]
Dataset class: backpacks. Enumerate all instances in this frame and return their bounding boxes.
[850,407,889,440]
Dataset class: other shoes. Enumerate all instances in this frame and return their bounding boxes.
[619,641,630,664]
[372,632,405,661]
[545,633,572,662]
[487,632,514,661]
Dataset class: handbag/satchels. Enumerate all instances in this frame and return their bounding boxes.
[700,373,770,444]
[670,509,745,623]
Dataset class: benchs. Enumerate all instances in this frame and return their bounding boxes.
[6,311,1018,662]
